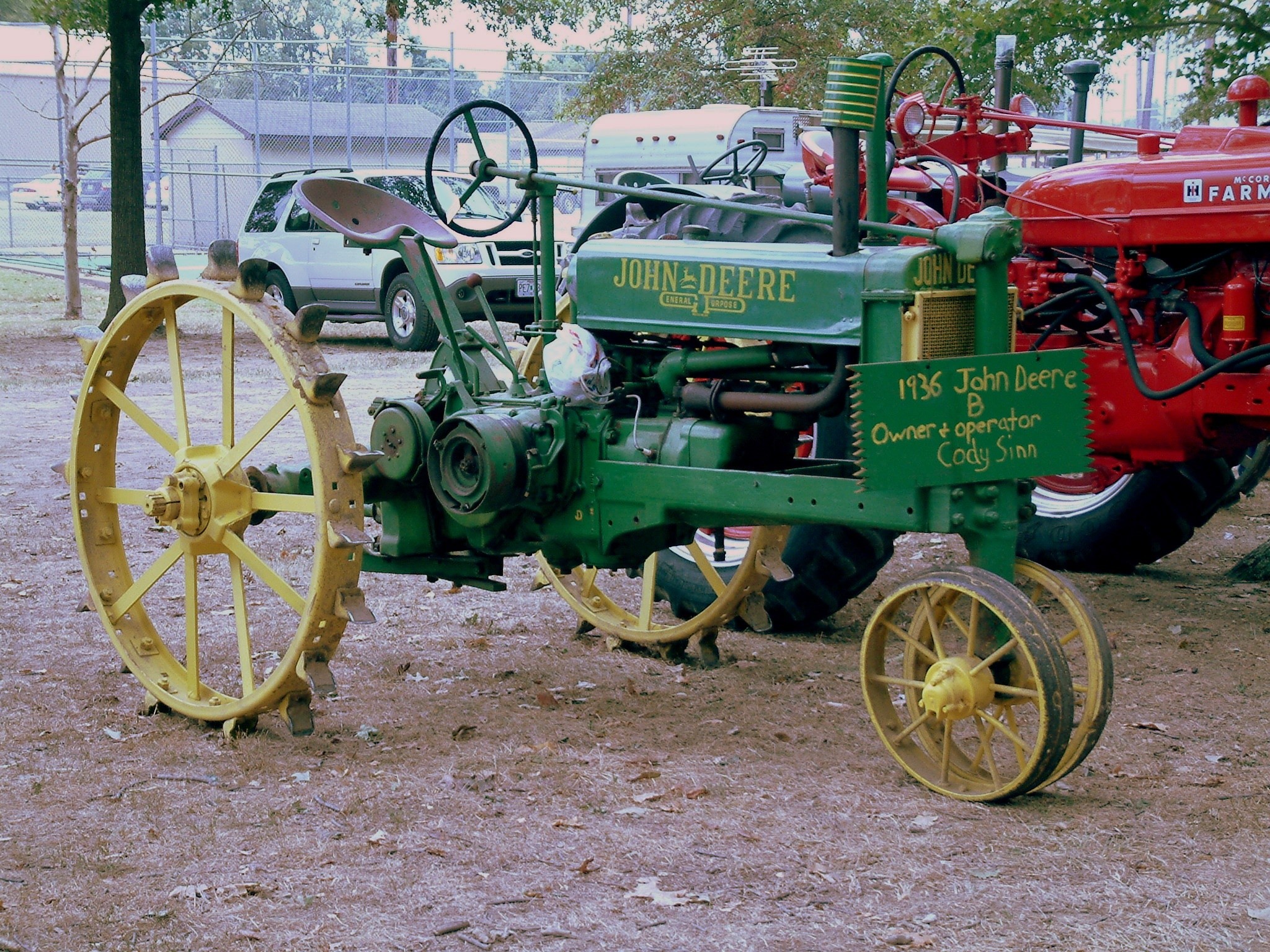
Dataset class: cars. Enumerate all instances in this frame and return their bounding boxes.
[12,173,62,212]
[478,162,578,215]
[77,162,172,211]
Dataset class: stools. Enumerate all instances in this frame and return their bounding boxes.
[796,127,934,205]
[610,160,675,207]
[291,173,461,249]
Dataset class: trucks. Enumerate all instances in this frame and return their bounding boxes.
[579,101,972,226]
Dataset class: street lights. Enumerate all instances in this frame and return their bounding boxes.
[539,76,561,123]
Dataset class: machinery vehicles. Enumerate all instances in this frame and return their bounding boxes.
[557,33,1270,634]
[51,49,1115,807]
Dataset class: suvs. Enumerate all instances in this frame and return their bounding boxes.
[236,166,562,351]
